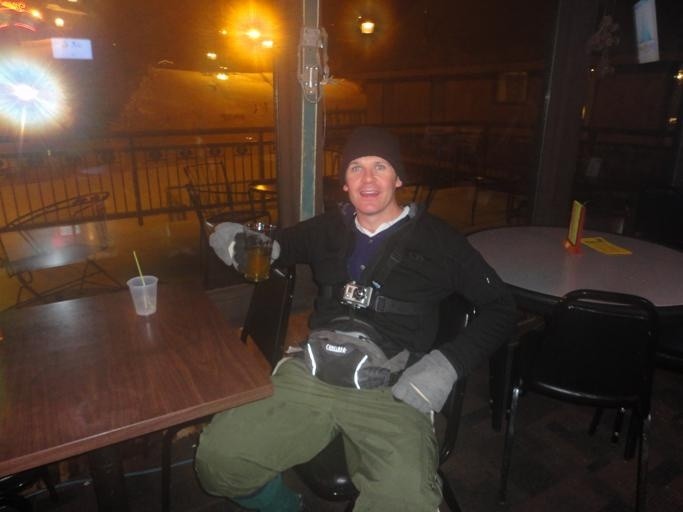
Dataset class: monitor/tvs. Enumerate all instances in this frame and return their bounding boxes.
[632,0,661,64]
[48,36,95,61]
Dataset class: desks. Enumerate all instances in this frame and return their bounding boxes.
[0,273,274,509]
[244,179,334,223]
[441,225,682,459]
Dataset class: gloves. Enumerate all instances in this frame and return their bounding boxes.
[391,348,458,417]
[207,220,281,274]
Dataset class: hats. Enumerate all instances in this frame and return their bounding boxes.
[340,127,402,185]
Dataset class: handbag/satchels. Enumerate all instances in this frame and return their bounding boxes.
[298,313,413,390]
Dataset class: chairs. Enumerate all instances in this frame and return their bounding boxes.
[291,291,477,508]
[472,265,659,508]
[0,190,126,304]
[158,257,295,508]
[184,161,269,250]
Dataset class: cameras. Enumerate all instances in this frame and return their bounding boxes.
[339,282,373,309]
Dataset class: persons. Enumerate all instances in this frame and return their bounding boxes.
[190,128,521,512]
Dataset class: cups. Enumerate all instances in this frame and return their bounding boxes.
[242,220,277,283]
[125,276,158,316]
